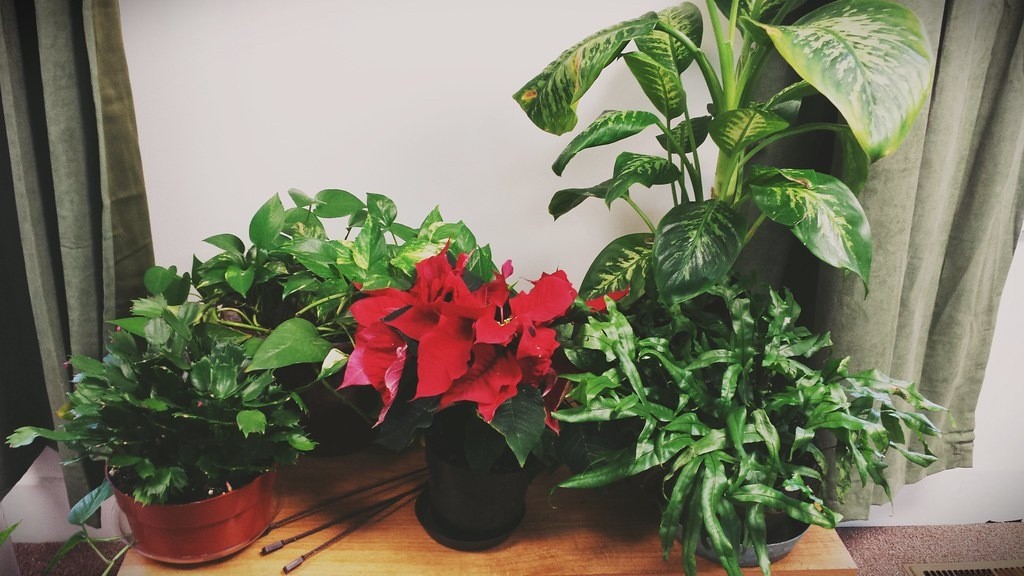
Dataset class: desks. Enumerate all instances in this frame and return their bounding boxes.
[115,436,861,576]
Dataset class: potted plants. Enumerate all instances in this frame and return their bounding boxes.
[550,285,952,576]
[336,244,636,553]
[144,191,501,458]
[2,292,318,564]
